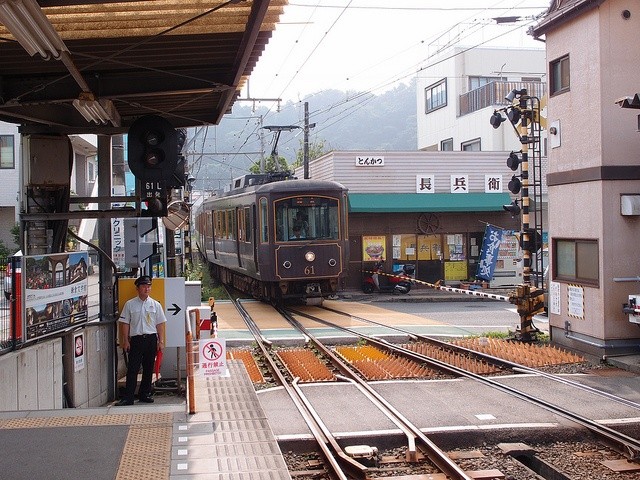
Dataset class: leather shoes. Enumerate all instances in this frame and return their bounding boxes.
[138,396,154,402]
[115,399,134,406]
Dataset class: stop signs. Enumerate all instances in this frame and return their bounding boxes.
[199,338,226,377]
[73,332,84,374]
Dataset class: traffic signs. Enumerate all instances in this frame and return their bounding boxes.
[119,277,186,347]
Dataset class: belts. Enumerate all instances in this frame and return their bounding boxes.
[139,334,156,338]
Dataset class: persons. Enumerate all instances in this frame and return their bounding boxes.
[294,211,309,237]
[114,276,167,406]
[288,226,305,240]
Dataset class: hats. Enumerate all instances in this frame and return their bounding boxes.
[134,276,152,285]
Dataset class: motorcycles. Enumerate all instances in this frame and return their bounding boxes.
[360,255,416,294]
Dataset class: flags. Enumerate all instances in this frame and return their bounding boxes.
[156,349,163,381]
[476,224,503,281]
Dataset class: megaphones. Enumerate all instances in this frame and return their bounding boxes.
[506,88,517,104]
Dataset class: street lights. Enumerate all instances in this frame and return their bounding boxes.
[503,201,520,215]
[262,103,309,180]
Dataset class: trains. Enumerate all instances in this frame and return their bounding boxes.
[193,171,349,307]
